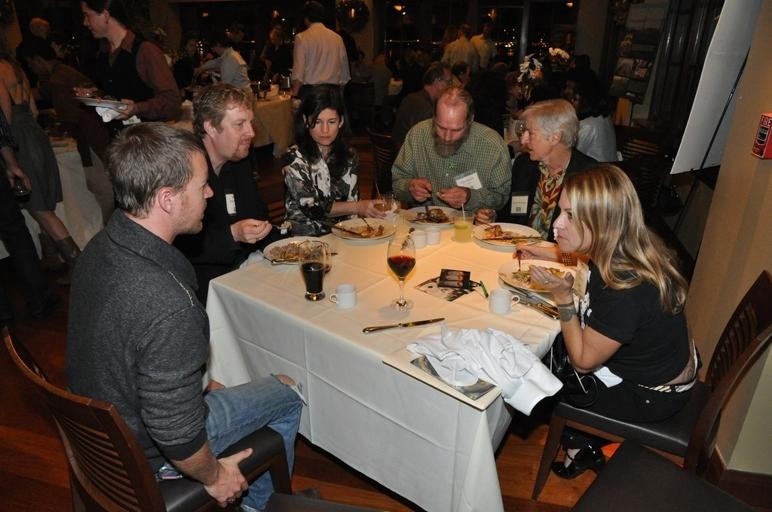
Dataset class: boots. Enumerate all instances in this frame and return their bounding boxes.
[55,236,81,285]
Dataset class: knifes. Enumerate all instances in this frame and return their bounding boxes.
[504,281,561,320]
[363,318,443,334]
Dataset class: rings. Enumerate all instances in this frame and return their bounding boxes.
[256,238,259,242]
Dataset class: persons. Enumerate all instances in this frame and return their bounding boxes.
[65,121,307,512]
[281,86,387,237]
[257,1,498,131]
[529,163,702,478]
[474,98,599,244]
[498,54,624,164]
[172,80,290,307]
[390,87,513,212]
[1,0,251,265]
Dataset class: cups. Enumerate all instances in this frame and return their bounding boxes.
[410,232,425,249]
[329,284,356,309]
[13,178,30,204]
[375,190,401,215]
[453,212,475,242]
[320,243,331,273]
[425,228,440,245]
[298,240,325,301]
[490,288,520,315]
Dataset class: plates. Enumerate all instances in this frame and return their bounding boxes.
[75,96,125,110]
[471,223,540,247]
[331,217,396,241]
[406,205,458,229]
[497,259,577,293]
[263,236,330,265]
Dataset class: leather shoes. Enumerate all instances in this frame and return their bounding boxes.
[30,293,64,320]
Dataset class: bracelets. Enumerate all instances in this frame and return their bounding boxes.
[557,301,574,307]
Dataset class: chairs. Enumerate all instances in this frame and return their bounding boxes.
[569,439,762,511]
[531,269,772,500]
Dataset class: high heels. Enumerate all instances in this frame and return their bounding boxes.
[551,443,606,480]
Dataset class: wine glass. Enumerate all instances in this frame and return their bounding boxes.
[514,122,526,137]
[386,237,416,313]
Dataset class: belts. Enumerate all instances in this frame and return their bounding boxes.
[637,338,698,393]
[301,83,340,88]
